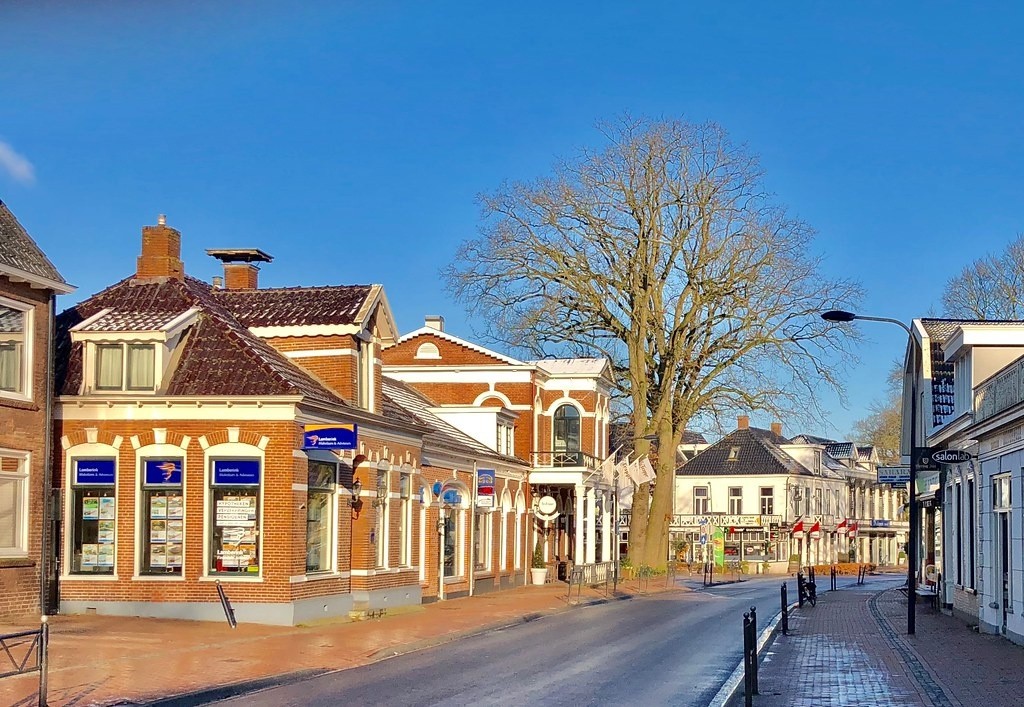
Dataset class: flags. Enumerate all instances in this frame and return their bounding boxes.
[837,518,849,533]
[616,456,657,489]
[809,521,820,540]
[848,523,859,539]
[602,454,615,486]
[793,521,803,538]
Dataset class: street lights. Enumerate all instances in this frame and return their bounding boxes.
[821,308,920,635]
[707,481,714,516]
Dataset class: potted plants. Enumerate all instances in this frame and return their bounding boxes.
[531,538,547,585]
[619,558,635,580]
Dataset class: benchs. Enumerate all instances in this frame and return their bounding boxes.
[915,573,940,612]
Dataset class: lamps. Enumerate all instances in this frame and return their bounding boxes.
[347,478,362,503]
[372,481,387,506]
